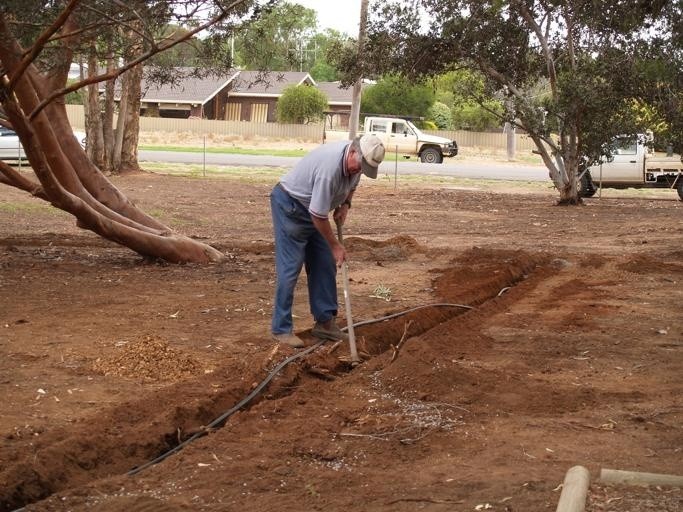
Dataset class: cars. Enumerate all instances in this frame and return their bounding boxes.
[0,124,86,161]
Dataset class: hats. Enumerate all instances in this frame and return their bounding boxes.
[359,134,385,178]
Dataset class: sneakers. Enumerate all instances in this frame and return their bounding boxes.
[271,331,305,347]
[310,316,356,341]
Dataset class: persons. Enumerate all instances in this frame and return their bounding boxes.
[270,133,386,348]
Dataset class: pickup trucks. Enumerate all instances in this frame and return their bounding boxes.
[548,130,682,203]
[322,117,458,165]
